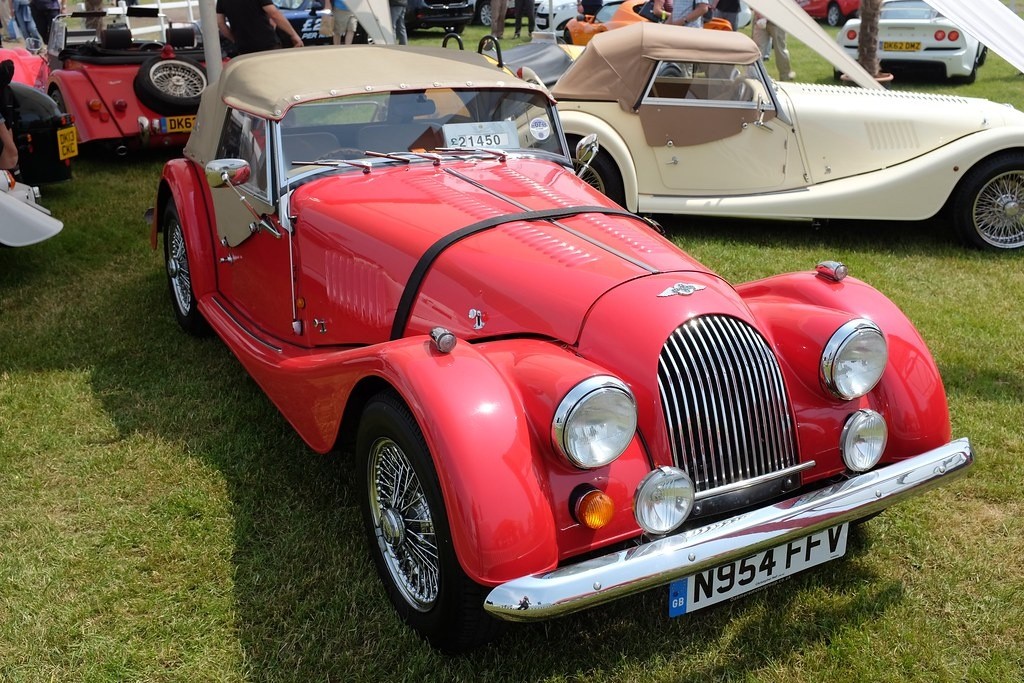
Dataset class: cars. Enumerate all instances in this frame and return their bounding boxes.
[151,47,975,657]
[494,20,1024,255]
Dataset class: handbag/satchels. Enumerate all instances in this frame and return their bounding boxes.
[693,0,712,24]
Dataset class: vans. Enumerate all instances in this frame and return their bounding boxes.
[834,0,989,84]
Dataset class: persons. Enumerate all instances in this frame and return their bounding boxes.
[215,0,305,51]
[388,0,407,45]
[0,0,105,47]
[322,0,356,45]
[0,114,19,171]
[490,0,536,40]
[577,0,602,14]
[752,12,796,82]
[653,0,741,77]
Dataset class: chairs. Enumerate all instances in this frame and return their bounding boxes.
[100,29,133,51]
[165,28,195,50]
[281,132,341,171]
[359,122,444,159]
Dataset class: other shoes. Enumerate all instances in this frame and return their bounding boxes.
[789,71,796,81]
[513,34,519,39]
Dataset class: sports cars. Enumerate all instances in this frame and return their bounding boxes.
[1,0,857,176]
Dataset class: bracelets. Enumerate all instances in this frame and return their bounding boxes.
[684,19,688,23]
